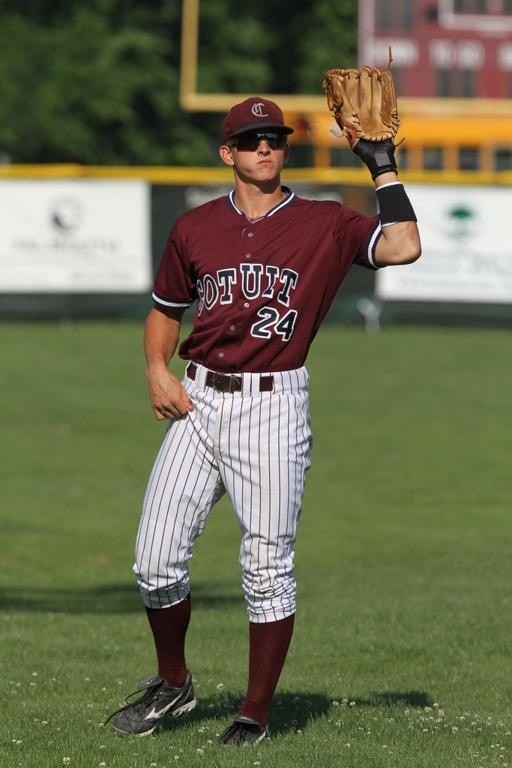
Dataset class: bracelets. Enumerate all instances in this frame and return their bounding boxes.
[374,180,418,229]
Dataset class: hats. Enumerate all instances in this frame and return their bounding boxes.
[221,97,293,145]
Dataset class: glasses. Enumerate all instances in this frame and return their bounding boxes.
[229,130,288,151]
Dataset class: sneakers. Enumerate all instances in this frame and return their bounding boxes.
[106,670,198,738]
[218,715,271,747]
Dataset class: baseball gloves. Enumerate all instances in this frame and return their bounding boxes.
[321,67,398,155]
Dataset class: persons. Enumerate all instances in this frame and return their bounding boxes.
[101,61,426,749]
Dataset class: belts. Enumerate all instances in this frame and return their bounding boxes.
[185,362,273,391]
